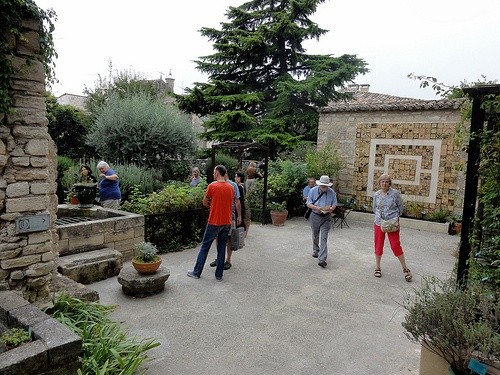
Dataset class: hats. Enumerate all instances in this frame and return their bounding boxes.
[315,175,334,187]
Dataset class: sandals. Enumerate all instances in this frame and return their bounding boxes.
[375,267,381,276]
[403,268,412,281]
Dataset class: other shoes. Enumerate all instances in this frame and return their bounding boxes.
[318,261,327,266]
[216,276,222,281]
[188,270,199,278]
[313,254,318,258]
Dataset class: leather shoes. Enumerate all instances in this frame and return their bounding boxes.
[210,258,232,270]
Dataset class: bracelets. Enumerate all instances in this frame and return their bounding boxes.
[104,175,106,178]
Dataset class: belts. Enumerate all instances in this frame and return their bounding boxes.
[313,212,330,217]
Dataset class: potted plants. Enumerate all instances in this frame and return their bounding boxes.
[132,241,162,274]
[0,327,32,352]
[267,201,288,226]
[72,172,100,209]
[57,155,72,203]
[450,213,462,233]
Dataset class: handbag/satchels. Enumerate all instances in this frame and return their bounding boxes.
[230,220,247,251]
[304,203,314,220]
[380,216,399,233]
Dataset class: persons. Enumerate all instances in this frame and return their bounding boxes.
[95,160,122,212]
[186,164,235,282]
[234,170,248,245]
[371,173,412,282]
[245,158,266,192]
[189,167,202,187]
[306,175,337,269]
[302,178,316,220]
[209,173,242,270]
[74,164,97,205]
